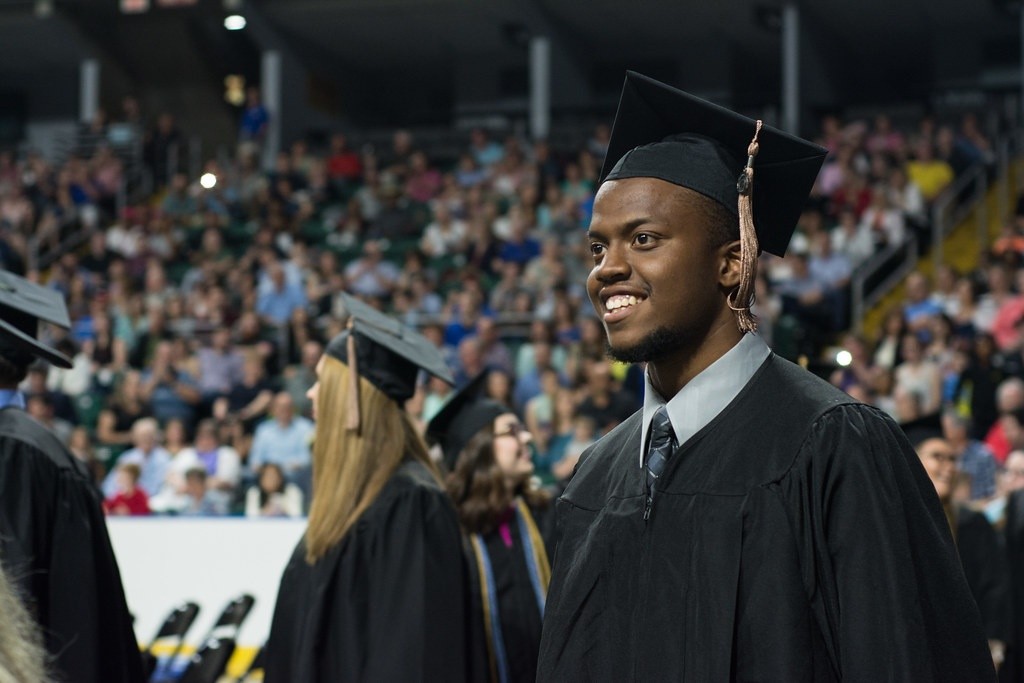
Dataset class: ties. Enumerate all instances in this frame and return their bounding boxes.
[645,405,674,493]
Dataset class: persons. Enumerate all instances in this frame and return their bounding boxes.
[422,367,555,683]
[264,285,485,683]
[532,69,997,683]
[1,0,1024,683]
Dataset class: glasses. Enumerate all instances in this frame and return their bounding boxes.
[492,427,521,437]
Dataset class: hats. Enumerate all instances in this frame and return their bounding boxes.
[1,321,73,370]
[424,366,513,472]
[0,270,71,339]
[898,412,946,448]
[324,292,455,436]
[595,69,828,334]
